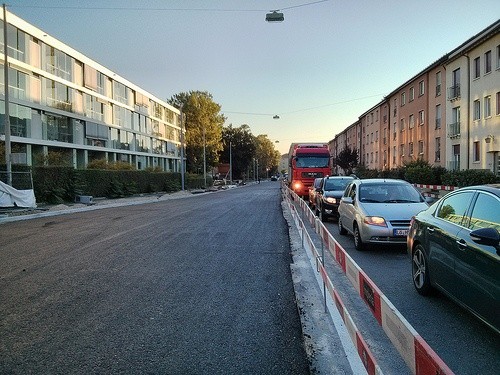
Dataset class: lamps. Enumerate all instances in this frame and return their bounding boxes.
[485,135,495,144]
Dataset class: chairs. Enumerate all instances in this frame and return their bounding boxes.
[361,190,369,199]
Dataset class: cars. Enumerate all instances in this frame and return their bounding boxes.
[271,172,288,184]
[307,178,323,210]
[337,179,433,251]
[211,178,243,191]
[406,186,500,336]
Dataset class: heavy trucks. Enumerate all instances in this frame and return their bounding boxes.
[285,143,333,201]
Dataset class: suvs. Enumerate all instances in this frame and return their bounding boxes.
[315,174,362,222]
